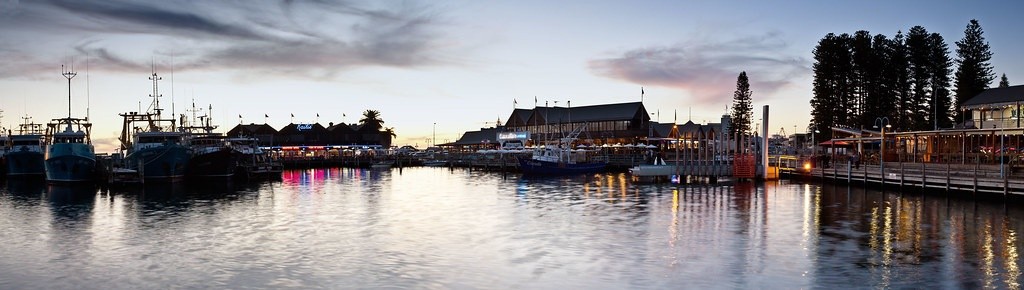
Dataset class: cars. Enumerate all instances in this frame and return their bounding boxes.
[995,147,1017,162]
[968,146,994,160]
[1010,148,1024,163]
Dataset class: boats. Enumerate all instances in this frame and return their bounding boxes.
[119,109,195,179]
[517,122,611,175]
[228,137,284,176]
[178,118,234,175]
[2,114,46,176]
[44,65,96,180]
[372,162,393,168]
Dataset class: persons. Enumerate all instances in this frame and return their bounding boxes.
[853,152,860,170]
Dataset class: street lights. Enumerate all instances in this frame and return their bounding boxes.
[988,106,1018,179]
[873,117,891,172]
[433,123,436,147]
[812,125,820,153]
